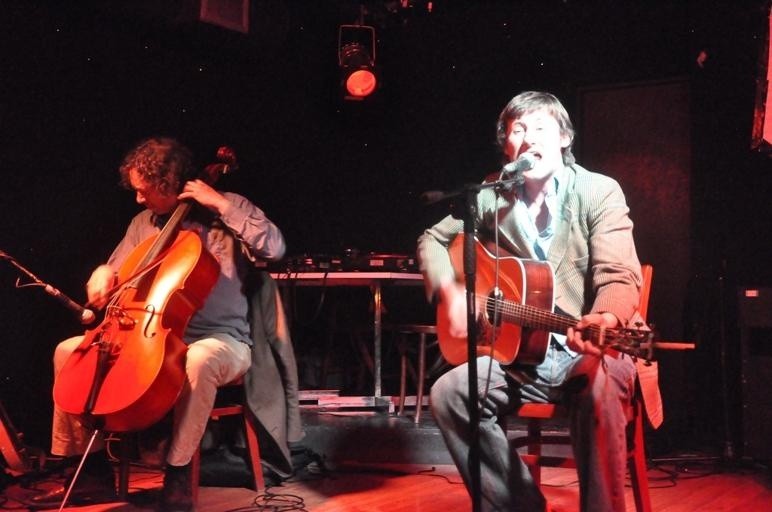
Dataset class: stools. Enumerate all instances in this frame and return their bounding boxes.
[396,324,451,424]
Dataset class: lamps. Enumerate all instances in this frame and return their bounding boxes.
[336,0,380,100]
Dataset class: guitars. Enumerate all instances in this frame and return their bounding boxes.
[437,233,655,366]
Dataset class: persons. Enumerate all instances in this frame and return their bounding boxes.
[26,132,286,511]
[413,88,642,511]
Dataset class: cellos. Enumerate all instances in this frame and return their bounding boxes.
[51,146,237,432]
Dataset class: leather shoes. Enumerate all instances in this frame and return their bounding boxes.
[21,466,115,508]
[160,464,195,512]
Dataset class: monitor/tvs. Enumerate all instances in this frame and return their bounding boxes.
[196,1,250,35]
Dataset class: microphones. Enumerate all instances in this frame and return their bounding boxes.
[45,284,96,324]
[502,151,535,177]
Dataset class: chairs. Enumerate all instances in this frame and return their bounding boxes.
[118,374,265,497]
[496,263,654,512]
